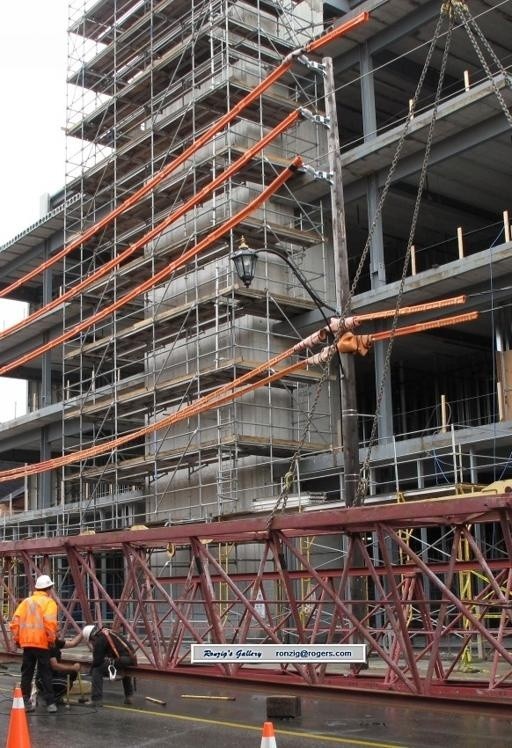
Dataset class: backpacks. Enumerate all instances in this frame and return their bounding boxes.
[114,656,137,677]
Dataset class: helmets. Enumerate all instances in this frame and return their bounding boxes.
[82,625,95,643]
[35,574,56,589]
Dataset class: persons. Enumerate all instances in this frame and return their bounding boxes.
[10,575,59,711]
[82,625,138,707]
[35,618,84,706]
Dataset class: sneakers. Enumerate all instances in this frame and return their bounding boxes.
[124,696,134,705]
[47,703,58,713]
[85,699,104,707]
[23,695,33,708]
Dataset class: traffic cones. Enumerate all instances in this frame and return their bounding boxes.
[260,721,277,748]
[6,687,33,748]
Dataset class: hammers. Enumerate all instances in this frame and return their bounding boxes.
[65,675,70,711]
[78,672,89,703]
